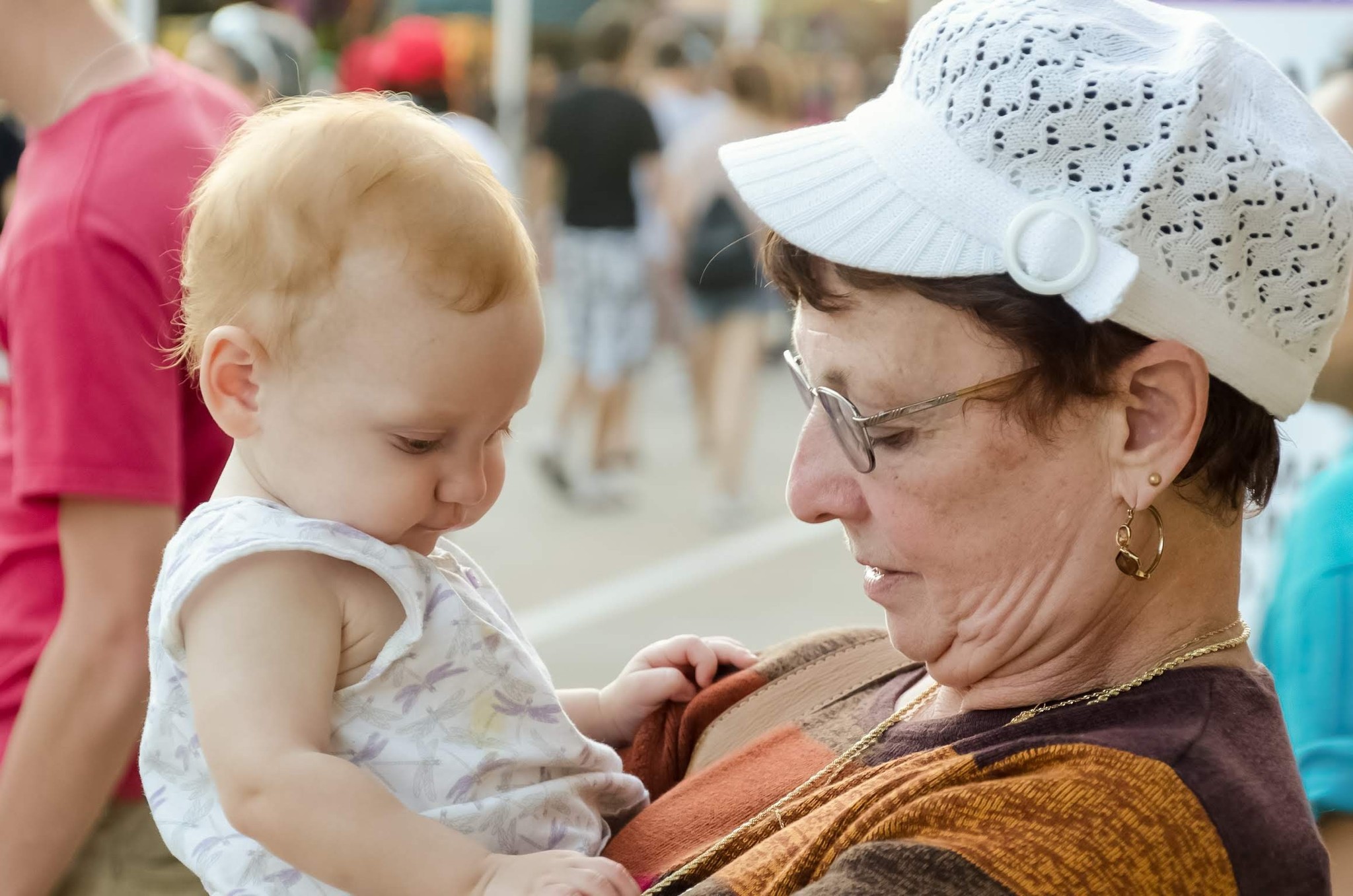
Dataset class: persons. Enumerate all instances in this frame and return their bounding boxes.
[1239,71,1352,896]
[137,89,759,896]
[0,0,508,896]
[523,1,779,492]
[600,1,1353,896]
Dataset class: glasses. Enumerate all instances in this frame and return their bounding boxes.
[785,350,1034,470]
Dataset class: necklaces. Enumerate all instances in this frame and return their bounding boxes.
[640,618,1250,896]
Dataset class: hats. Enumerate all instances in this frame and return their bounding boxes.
[721,0,1353,432]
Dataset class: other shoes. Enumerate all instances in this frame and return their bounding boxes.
[535,446,574,498]
